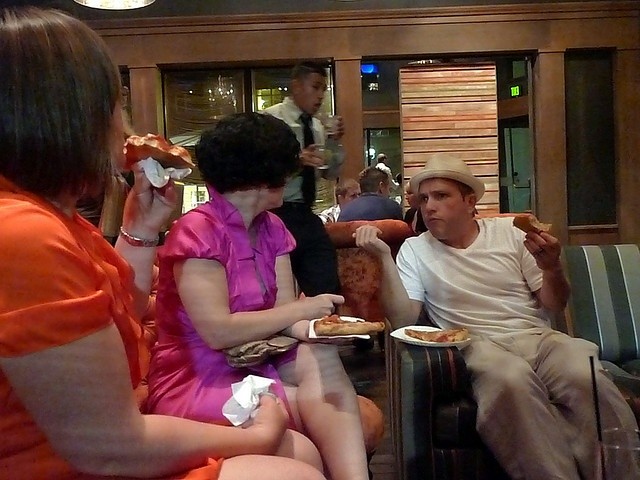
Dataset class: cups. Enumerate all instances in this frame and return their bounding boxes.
[315,147,332,169]
[324,114,340,134]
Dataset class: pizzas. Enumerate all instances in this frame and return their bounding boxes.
[405,328,470,343]
[314,314,385,336]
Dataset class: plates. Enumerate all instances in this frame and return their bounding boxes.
[389,325,470,347]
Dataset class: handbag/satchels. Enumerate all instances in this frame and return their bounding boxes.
[222,333,300,367]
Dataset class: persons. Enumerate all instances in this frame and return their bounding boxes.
[318,176,362,225]
[372,153,399,197]
[147,111,370,480]
[336,167,403,221]
[266,61,343,362]
[0,5,328,480]
[352,149,639,480]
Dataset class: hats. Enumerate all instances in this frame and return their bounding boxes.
[409,156,487,198]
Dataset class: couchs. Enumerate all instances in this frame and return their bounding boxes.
[311,219,412,326]
[386,244,640,475]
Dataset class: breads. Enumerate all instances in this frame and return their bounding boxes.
[513,213,553,233]
[122,133,196,170]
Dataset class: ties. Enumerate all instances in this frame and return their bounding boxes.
[301,116,315,205]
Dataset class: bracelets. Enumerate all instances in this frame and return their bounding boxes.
[119,226,161,249]
